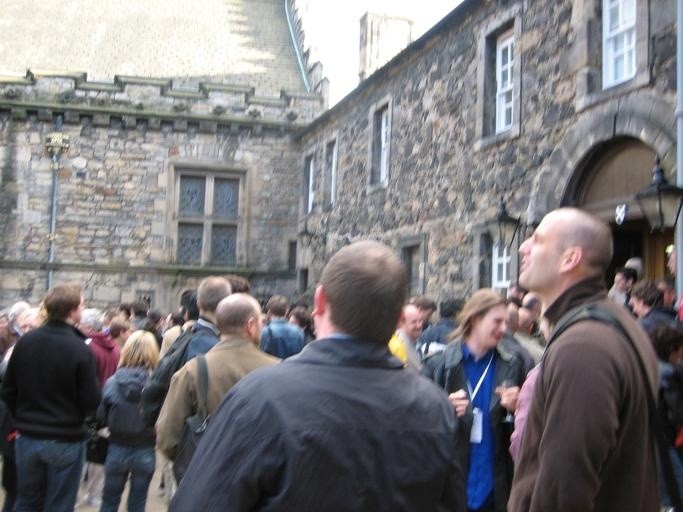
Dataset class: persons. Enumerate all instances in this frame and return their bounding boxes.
[0,206,683,512]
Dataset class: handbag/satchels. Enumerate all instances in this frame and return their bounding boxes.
[172,416,208,489]
[84,433,109,465]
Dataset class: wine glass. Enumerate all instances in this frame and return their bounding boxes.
[500,379,517,423]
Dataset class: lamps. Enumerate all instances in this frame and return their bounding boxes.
[632,150,681,237]
[483,193,526,256]
